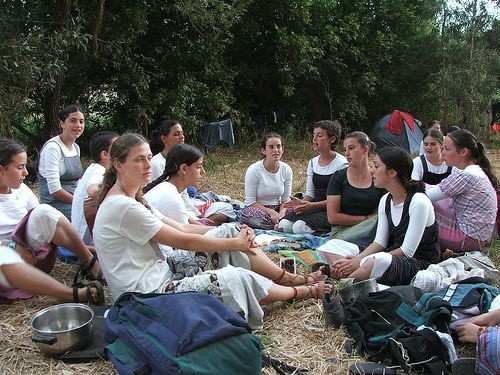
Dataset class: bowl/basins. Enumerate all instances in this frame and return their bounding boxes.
[339,278,378,302]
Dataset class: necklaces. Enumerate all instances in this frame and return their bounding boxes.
[316,154,336,175]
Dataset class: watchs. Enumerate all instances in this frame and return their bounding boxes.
[477,326,486,335]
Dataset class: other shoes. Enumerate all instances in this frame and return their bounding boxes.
[312,262,343,278]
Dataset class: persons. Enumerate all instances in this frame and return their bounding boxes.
[240,133,303,231]
[0,139,107,305]
[326,131,389,249]
[316,147,441,286]
[71,119,333,330]
[36,104,85,222]
[411,120,499,251]
[451,309,500,375]
[279,120,349,235]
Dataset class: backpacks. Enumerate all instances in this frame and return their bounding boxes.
[342,276,494,375]
[105,291,265,375]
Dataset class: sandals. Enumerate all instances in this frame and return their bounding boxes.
[286,281,334,304]
[274,267,328,286]
[74,254,107,286]
[71,282,104,306]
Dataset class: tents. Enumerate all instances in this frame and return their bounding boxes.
[368,110,425,159]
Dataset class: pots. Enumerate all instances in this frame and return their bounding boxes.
[30,302,95,356]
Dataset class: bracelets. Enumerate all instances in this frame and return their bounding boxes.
[364,215,369,220]
[8,240,16,250]
